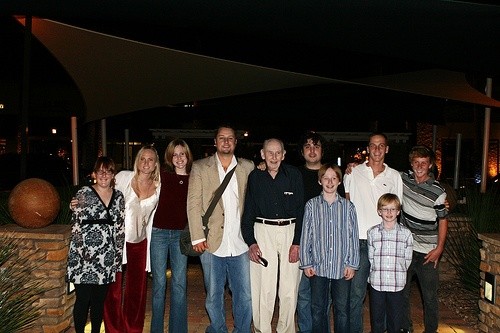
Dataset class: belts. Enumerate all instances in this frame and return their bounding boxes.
[254,219,296,226]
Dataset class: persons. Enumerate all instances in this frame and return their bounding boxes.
[69,144,162,333]
[349,145,448,333]
[240,138,307,333]
[186,127,254,333]
[64,157,125,333]
[143,138,195,333]
[344,132,450,333]
[367,193,414,333]
[256,130,345,333]
[296,163,360,333]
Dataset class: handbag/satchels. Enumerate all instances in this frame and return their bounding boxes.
[180,223,209,256]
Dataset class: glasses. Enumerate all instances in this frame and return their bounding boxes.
[96,170,113,175]
[257,256,268,267]
[380,208,400,212]
